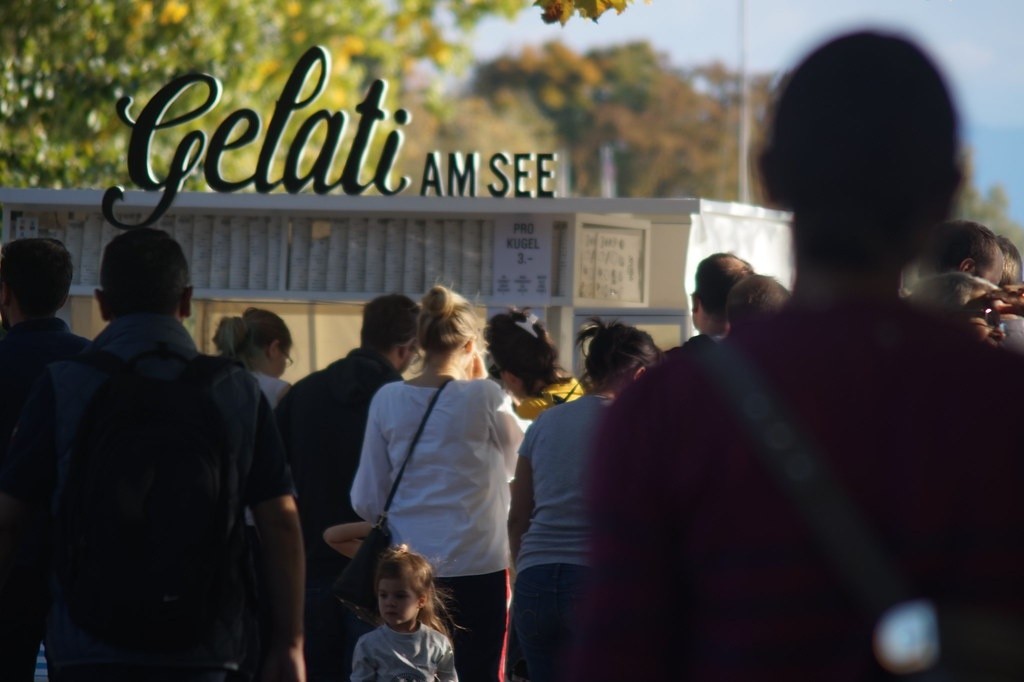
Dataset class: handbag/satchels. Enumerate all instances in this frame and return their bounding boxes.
[334,527,394,627]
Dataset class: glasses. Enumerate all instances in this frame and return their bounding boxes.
[489,364,505,381]
[954,305,999,328]
[280,349,293,367]
[397,343,421,365]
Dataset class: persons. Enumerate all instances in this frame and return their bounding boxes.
[907,219,1023,351]
[606,28,1024,682]
[1,227,792,682]
